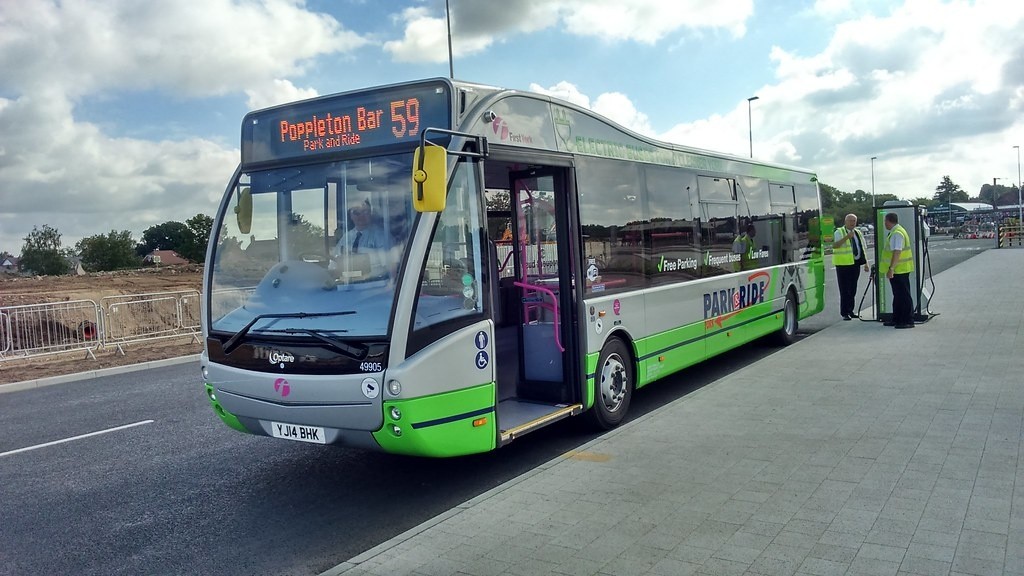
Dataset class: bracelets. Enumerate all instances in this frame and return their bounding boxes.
[889,267,894,270]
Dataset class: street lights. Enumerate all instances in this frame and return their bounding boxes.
[686,186,693,220]
[870,156,880,209]
[747,97,760,157]
[1013,145,1024,234]
[778,183,795,205]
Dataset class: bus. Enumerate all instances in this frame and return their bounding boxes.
[820,215,834,242]
[199,78,826,461]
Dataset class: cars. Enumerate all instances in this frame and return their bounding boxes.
[855,224,874,235]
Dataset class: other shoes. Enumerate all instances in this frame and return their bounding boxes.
[842,315,851,320]
[895,323,914,328]
[883,319,896,326]
[851,314,859,318]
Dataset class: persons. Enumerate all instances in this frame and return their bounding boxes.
[960,216,997,239]
[882,213,915,329]
[328,199,397,278]
[732,225,760,269]
[832,214,870,320]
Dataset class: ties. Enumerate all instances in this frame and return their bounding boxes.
[846,229,858,256]
[887,233,890,236]
[352,232,362,255]
[751,239,753,243]
